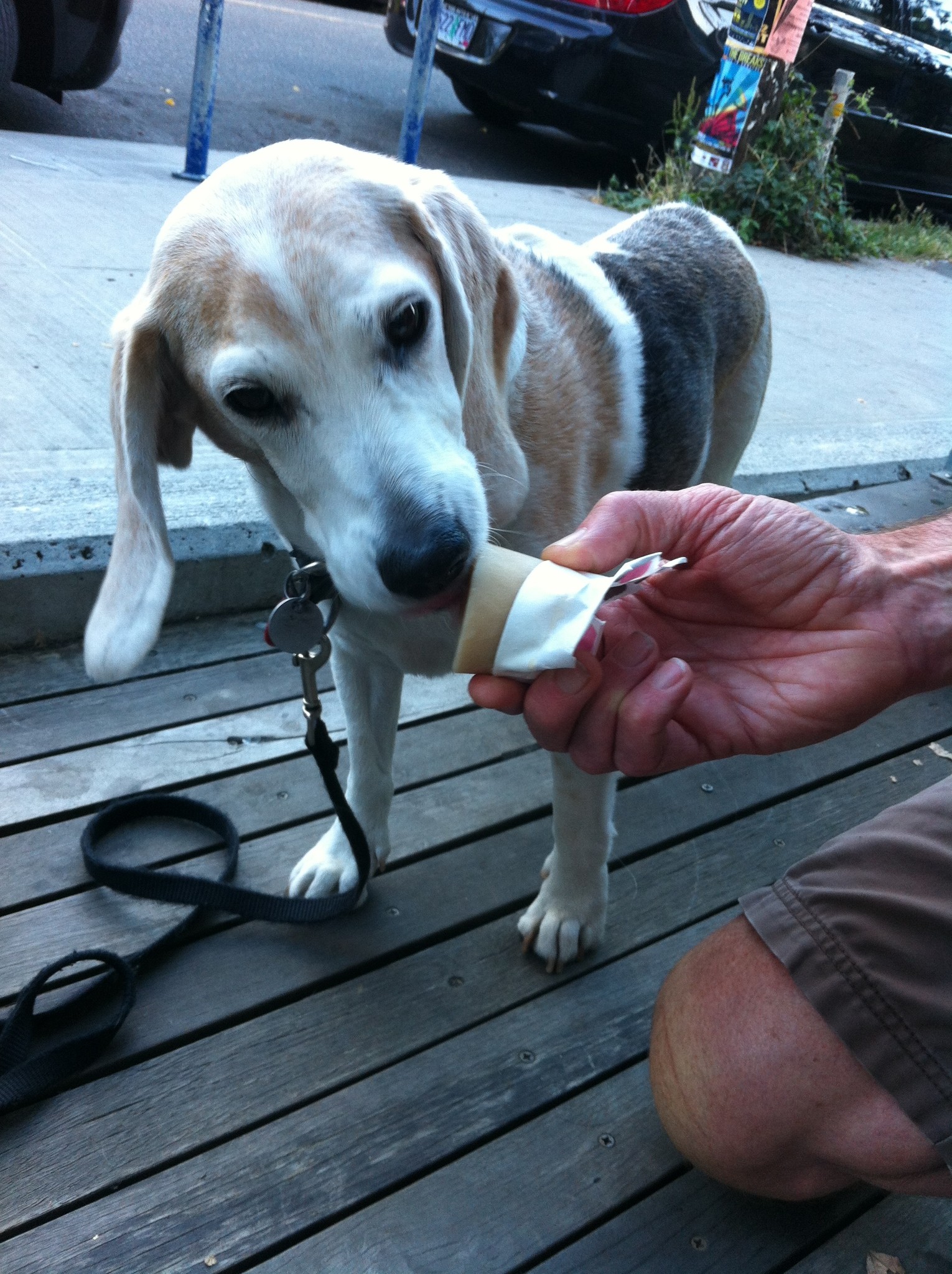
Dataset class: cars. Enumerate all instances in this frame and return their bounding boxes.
[382,2,951,215]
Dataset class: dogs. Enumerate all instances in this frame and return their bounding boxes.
[81,139,774,974]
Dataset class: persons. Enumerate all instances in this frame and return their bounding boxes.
[477,488,952,1206]
